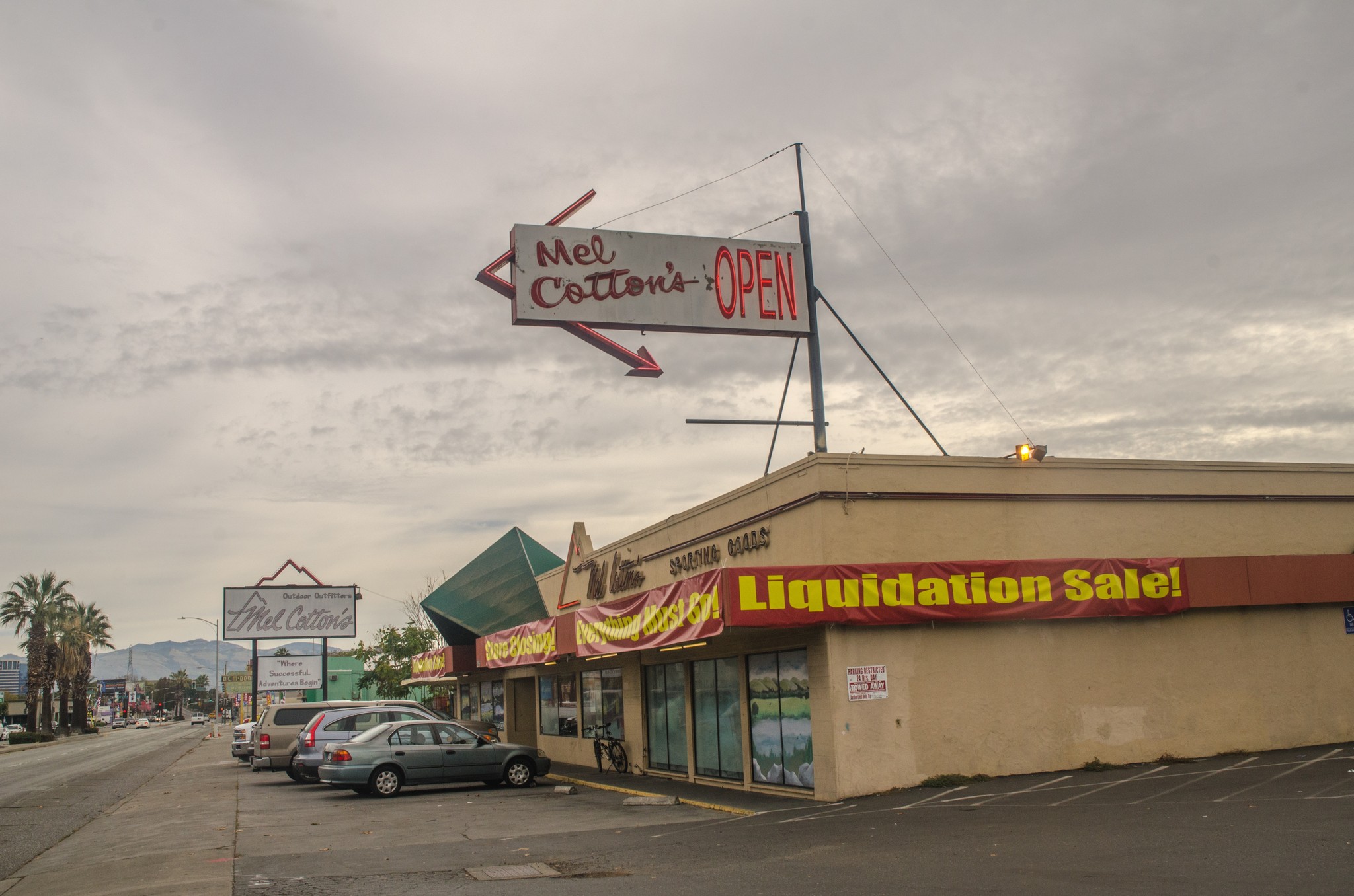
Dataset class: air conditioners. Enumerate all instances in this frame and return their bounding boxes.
[329,675,338,680]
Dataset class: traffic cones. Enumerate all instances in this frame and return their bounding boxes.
[217,730,221,737]
[205,732,211,737]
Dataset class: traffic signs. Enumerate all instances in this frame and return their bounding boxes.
[473,189,810,379]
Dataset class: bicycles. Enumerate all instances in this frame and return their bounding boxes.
[581,723,628,776]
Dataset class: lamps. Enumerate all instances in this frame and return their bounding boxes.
[356,587,363,600]
[1003,444,1047,462]
[454,669,469,677]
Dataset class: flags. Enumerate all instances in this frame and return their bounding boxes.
[280,691,285,704]
[97,693,101,706]
[266,691,275,706]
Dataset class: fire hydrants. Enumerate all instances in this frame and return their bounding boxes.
[229,721,231,726]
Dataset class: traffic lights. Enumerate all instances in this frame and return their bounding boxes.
[159,703,162,706]
[222,683,225,693]
[210,699,212,702]
[224,698,227,705]
[191,681,196,689]
[123,709,126,713]
[221,707,224,711]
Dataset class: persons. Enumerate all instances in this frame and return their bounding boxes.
[127,705,132,712]
[131,694,134,702]
[117,705,119,711]
[232,714,234,722]
[134,684,141,691]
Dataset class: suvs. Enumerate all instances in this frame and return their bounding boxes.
[0,719,58,742]
[561,697,663,734]
[112,718,127,729]
[291,706,501,774]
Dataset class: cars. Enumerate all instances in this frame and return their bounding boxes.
[318,720,552,799]
[231,712,262,761]
[135,719,150,728]
[127,711,172,725]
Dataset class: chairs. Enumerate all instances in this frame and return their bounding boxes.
[417,733,426,745]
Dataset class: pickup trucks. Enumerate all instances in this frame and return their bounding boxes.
[203,713,215,721]
[191,712,205,725]
[249,699,499,781]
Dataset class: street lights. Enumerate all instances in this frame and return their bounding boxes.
[151,688,169,716]
[177,617,219,738]
[164,692,175,709]
[196,667,224,699]
